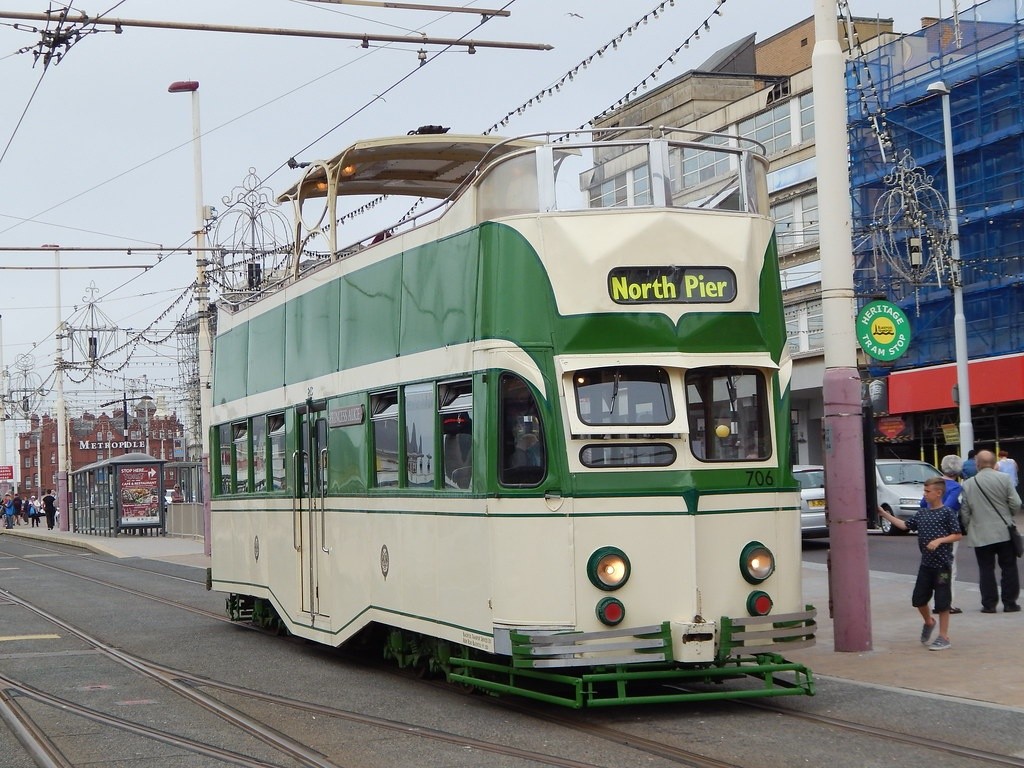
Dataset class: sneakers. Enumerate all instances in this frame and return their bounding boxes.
[920,617,936,642]
[928,636,951,650]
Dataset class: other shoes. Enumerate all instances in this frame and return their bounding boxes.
[1004,604,1020,611]
[981,606,996,613]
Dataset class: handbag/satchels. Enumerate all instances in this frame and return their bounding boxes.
[1008,523,1024,558]
[14,500,19,508]
[30,507,36,516]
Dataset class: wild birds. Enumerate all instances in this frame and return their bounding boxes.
[407,125,452,135]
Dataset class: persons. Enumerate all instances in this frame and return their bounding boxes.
[878,477,962,650]
[960,450,1022,613]
[160,484,184,534]
[0,489,57,530]
[919,455,963,614]
[962,450,1019,493]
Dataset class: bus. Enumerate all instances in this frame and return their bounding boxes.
[210,122,819,714]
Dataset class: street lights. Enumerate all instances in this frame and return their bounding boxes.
[31,435,41,503]
[101,396,154,453]
[929,80,974,456]
[169,81,214,553]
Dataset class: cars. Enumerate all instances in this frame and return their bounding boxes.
[875,458,963,534]
[21,495,60,522]
[791,464,828,536]
[165,489,186,511]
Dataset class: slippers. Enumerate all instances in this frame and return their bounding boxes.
[932,604,963,613]
